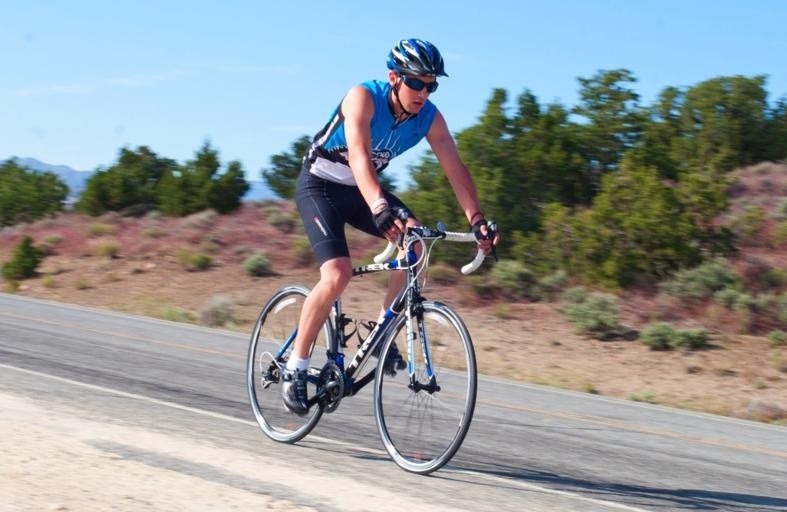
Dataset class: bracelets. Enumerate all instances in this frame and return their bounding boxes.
[370,198,389,212]
[471,212,485,225]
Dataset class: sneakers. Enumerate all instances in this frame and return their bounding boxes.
[371,341,407,370]
[282,364,308,414]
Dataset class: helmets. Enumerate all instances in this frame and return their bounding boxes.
[386,39,450,77]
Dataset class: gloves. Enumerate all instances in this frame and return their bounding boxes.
[472,219,498,245]
[372,206,409,235]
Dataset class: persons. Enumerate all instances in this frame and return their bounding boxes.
[281,38,503,415]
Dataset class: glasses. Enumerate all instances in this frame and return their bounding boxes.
[401,76,439,93]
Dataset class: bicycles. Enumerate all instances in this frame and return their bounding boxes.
[246,205,500,476]
